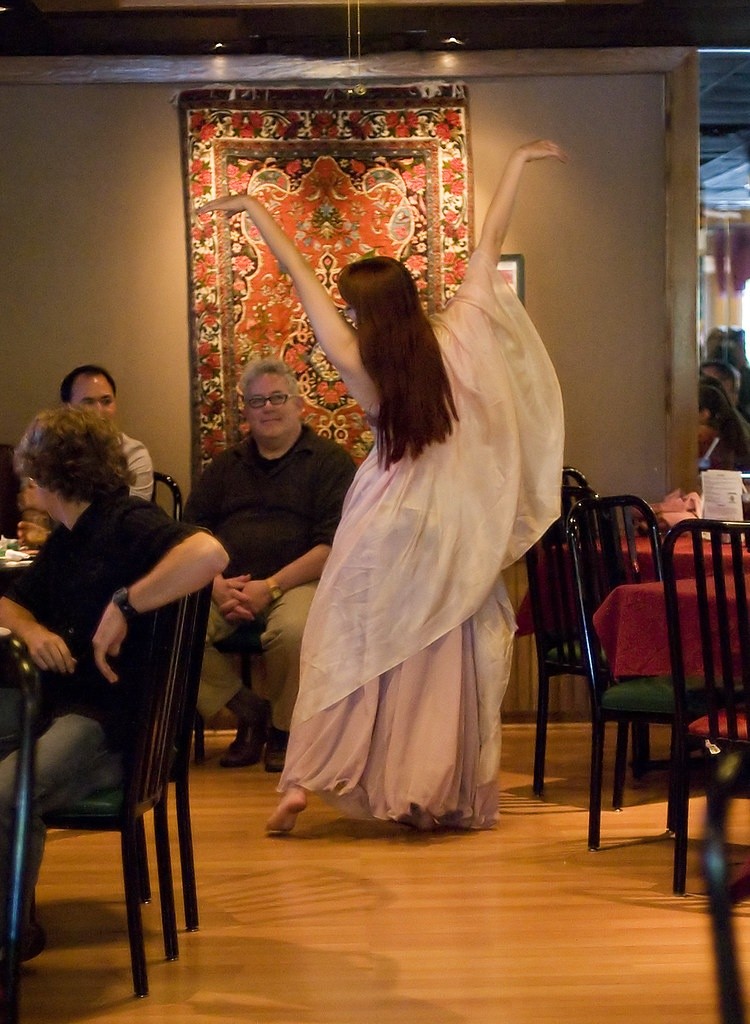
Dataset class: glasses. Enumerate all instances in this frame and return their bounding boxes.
[243,394,300,408]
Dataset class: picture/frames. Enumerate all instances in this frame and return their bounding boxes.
[497,254,525,301]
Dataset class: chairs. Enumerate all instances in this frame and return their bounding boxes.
[521,468,750,1024]
[0,471,215,1024]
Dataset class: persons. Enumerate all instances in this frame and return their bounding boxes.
[698,325,750,474]
[199,139,568,833]
[182,360,358,773]
[0,364,230,962]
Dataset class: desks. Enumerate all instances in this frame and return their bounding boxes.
[594,567,750,681]
[512,539,750,640]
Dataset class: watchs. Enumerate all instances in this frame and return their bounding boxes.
[112,587,137,623]
[265,577,283,600]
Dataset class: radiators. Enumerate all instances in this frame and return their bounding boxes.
[497,562,593,715]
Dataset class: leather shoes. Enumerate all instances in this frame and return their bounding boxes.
[219,720,261,767]
[265,736,287,773]
